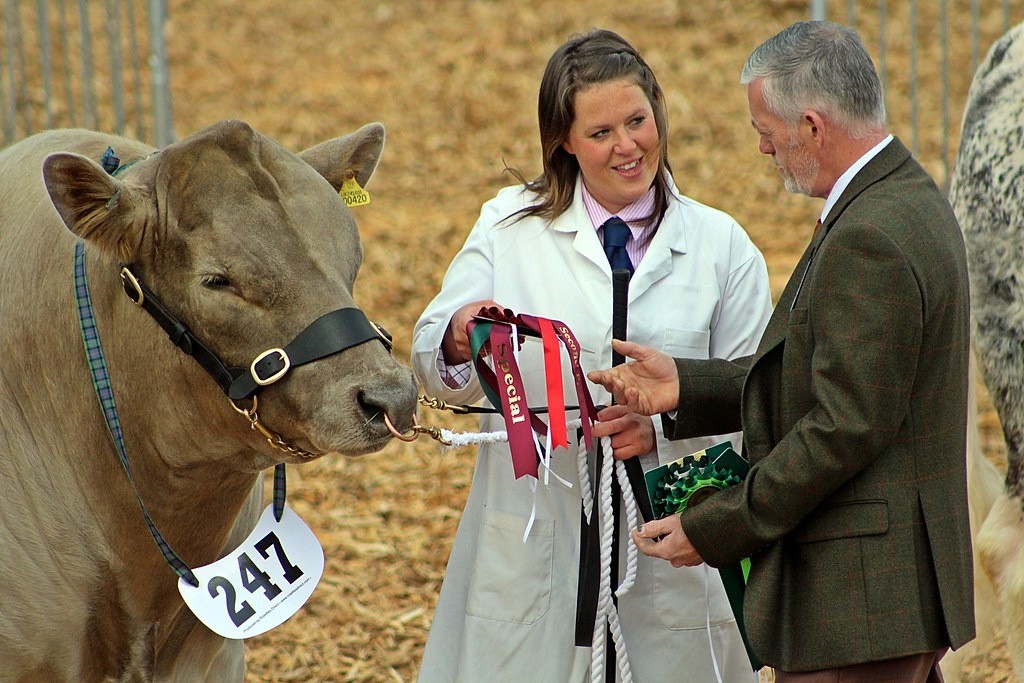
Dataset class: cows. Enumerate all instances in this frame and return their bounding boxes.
[940,22,1024,683]
[0,118,423,683]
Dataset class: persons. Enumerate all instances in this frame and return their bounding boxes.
[587,19,978,682]
[412,27,774,683]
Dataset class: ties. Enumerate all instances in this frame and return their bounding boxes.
[811,218,821,239]
[600,217,636,285]
